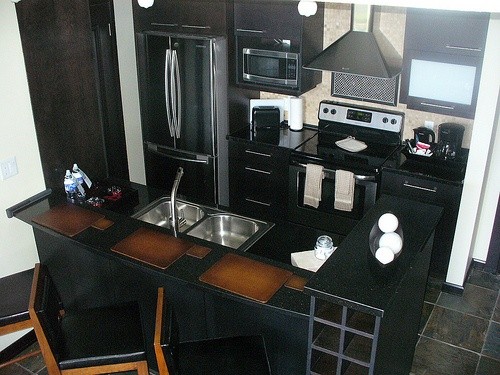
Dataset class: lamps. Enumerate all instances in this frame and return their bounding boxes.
[138,0,154,9]
[297,0,318,18]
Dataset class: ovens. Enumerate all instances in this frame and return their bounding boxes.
[285,160,380,254]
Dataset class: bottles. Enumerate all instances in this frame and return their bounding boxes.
[71,164,87,197]
[64,169,79,204]
[314,235,334,260]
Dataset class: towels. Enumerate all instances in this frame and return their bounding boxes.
[303,164,324,209]
[334,169,355,211]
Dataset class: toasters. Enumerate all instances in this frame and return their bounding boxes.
[252,105,280,129]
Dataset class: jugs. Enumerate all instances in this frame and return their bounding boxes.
[413,126,435,149]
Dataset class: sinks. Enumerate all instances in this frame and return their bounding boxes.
[134,199,206,233]
[186,212,260,251]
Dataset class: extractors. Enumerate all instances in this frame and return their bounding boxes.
[301,4,403,80]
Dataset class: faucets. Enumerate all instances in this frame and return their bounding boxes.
[169,165,187,235]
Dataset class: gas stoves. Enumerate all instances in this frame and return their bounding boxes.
[290,99,405,177]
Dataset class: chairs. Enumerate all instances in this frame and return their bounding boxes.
[154,287,272,375]
[0,268,42,368]
[29,263,149,375]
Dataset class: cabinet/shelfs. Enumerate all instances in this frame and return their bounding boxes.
[233,0,325,96]
[227,141,291,222]
[13,0,129,185]
[306,296,380,375]
[382,172,463,290]
[399,8,490,119]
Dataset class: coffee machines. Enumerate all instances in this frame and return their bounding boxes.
[437,123,465,159]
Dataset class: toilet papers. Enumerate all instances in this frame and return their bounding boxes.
[287,97,304,130]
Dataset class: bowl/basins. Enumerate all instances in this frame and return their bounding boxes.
[368,211,404,268]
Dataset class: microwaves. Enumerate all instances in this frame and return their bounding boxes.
[241,46,299,90]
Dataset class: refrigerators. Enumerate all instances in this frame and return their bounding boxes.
[135,29,228,212]
